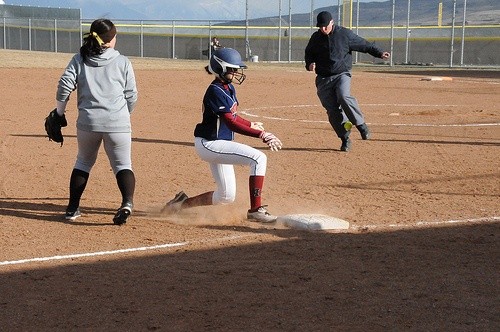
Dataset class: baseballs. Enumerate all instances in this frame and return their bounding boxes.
[344,121,353,131]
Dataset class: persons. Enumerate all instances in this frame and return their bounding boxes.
[211,37,221,55]
[160,47,282,223]
[45,19,138,225]
[305,11,390,152]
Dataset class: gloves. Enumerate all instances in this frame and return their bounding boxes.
[261,132,283,152]
[250,121,265,132]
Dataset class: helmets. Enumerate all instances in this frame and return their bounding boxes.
[208,48,248,85]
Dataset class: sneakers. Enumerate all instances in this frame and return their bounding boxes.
[159,191,188,214]
[65,211,81,219]
[341,136,350,152]
[356,123,369,140]
[247,205,277,223]
[113,202,133,224]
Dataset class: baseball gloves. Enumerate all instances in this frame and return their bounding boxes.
[43,108,67,148]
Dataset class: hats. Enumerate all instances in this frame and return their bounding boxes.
[316,11,332,27]
